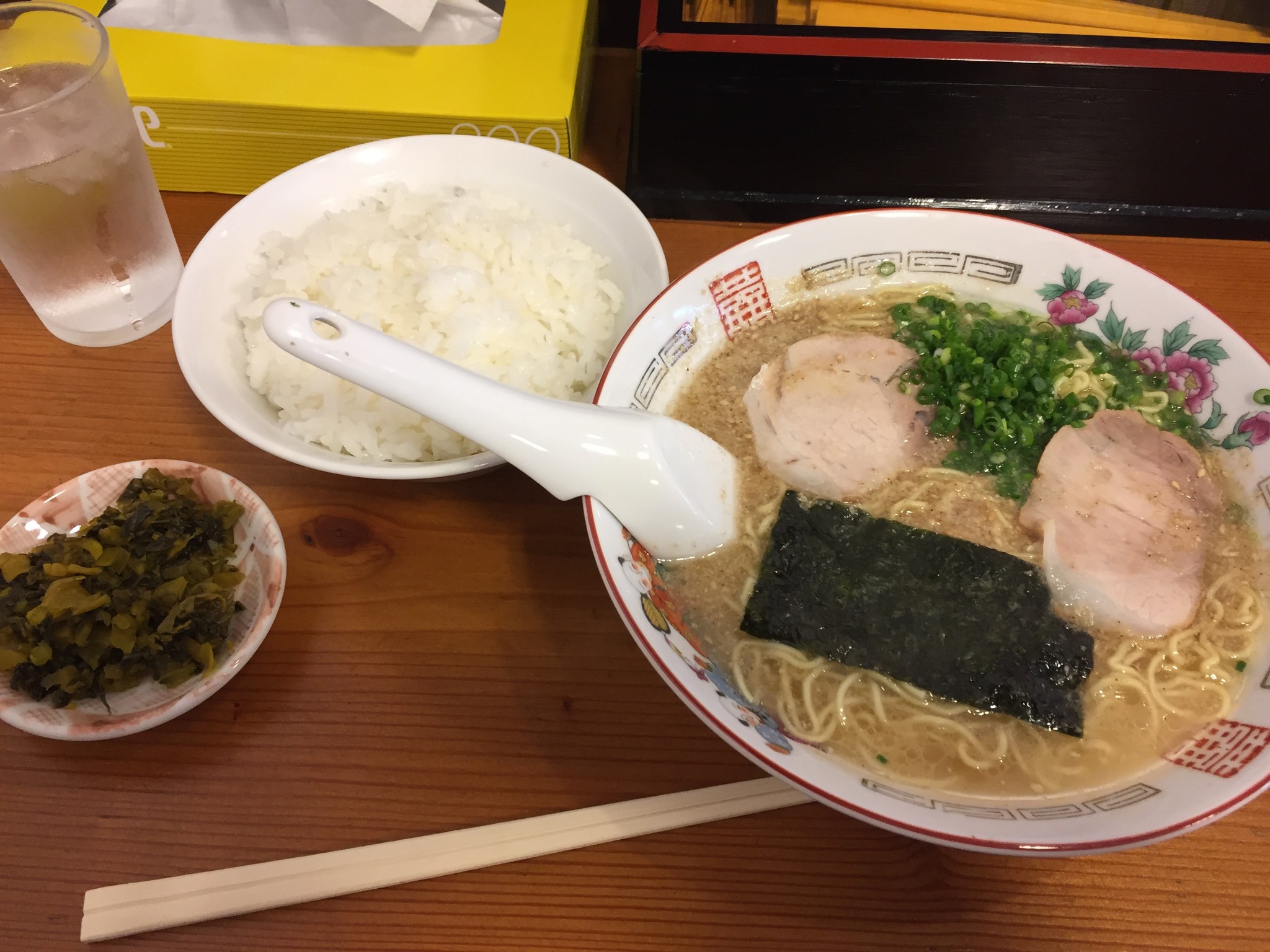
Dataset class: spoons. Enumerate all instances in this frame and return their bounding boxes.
[259,291,738,564]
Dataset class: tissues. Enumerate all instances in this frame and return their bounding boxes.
[0,0,601,197]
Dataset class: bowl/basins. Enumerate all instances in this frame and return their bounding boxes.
[580,205,1269,862]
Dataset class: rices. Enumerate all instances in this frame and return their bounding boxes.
[232,180,627,461]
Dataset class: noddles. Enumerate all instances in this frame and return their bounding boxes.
[643,279,1267,797]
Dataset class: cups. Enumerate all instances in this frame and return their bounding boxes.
[2,1,191,353]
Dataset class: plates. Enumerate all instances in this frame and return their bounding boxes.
[172,130,673,487]
[0,457,287,746]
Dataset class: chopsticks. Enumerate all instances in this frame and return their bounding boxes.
[75,768,828,945]
